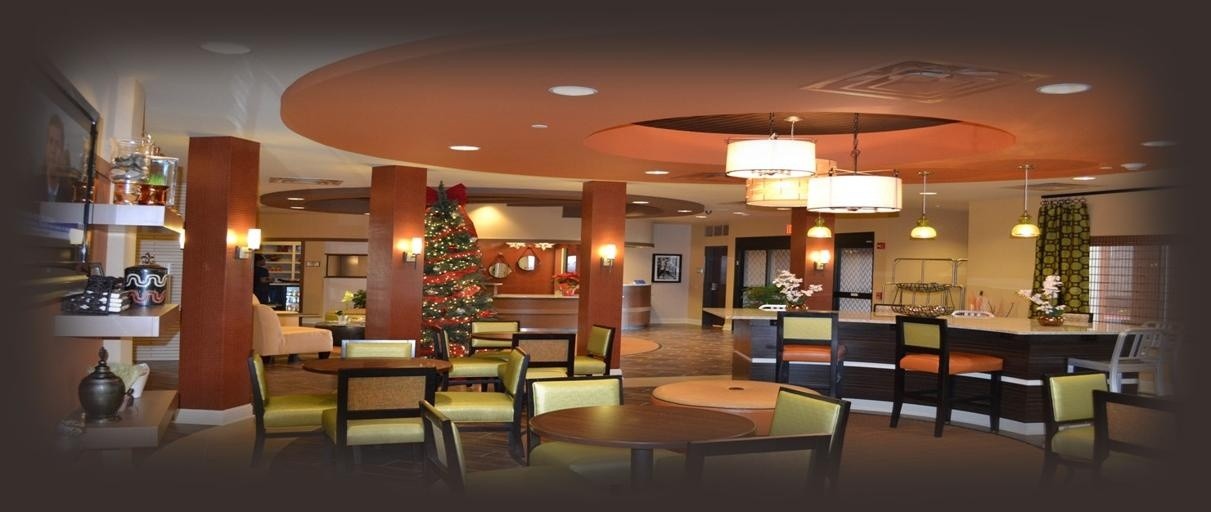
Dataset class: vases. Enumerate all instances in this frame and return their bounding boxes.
[1039,313,1064,327]
[786,302,808,313]
[560,282,579,296]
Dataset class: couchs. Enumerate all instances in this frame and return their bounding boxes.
[252,294,334,364]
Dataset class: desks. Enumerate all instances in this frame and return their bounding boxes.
[299,356,455,461]
[526,403,758,511]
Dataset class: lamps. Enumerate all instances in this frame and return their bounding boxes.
[400,237,423,270]
[1010,164,1043,238]
[740,116,837,210]
[233,226,265,262]
[805,215,837,240]
[726,111,818,181]
[908,168,940,241]
[804,113,902,214]
[600,243,620,271]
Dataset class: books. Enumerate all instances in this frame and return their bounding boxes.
[79,289,131,313]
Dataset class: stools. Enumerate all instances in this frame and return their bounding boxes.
[651,377,823,437]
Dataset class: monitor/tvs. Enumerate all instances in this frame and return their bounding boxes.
[0,22,103,265]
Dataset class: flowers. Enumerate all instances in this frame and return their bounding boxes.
[1017,273,1067,315]
[553,270,582,287]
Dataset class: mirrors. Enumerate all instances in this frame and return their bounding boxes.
[486,262,513,280]
[516,255,541,272]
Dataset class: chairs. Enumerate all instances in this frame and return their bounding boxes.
[1063,323,1171,393]
[657,385,853,511]
[321,365,439,469]
[566,321,618,378]
[429,323,506,394]
[431,347,532,463]
[342,336,420,363]
[247,354,336,471]
[1088,383,1197,510]
[773,307,849,400]
[1036,370,1120,494]
[675,433,830,512]
[464,319,522,359]
[416,398,594,512]
[890,313,1006,438]
[526,371,682,488]
[504,330,578,383]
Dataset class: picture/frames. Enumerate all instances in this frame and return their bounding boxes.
[773,269,824,304]
[652,252,682,284]
[14,48,104,310]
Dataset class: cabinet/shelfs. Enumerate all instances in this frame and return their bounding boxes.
[257,242,301,284]
[39,201,189,450]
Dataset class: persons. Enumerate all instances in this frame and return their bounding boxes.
[39,115,81,204]
[253,253,276,304]
